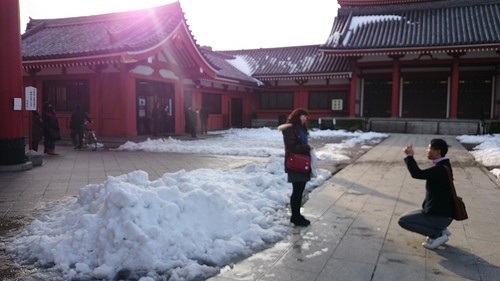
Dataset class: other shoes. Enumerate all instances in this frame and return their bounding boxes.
[290,214,311,227]
[422,225,453,252]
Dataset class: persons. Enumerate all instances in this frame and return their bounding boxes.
[32,101,61,155]
[398,138,456,249]
[152,101,208,138]
[278,109,314,226]
[69,104,93,149]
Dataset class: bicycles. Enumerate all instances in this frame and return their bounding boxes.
[81,120,98,152]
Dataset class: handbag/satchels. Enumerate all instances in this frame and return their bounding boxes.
[286,151,312,172]
[452,197,468,221]
